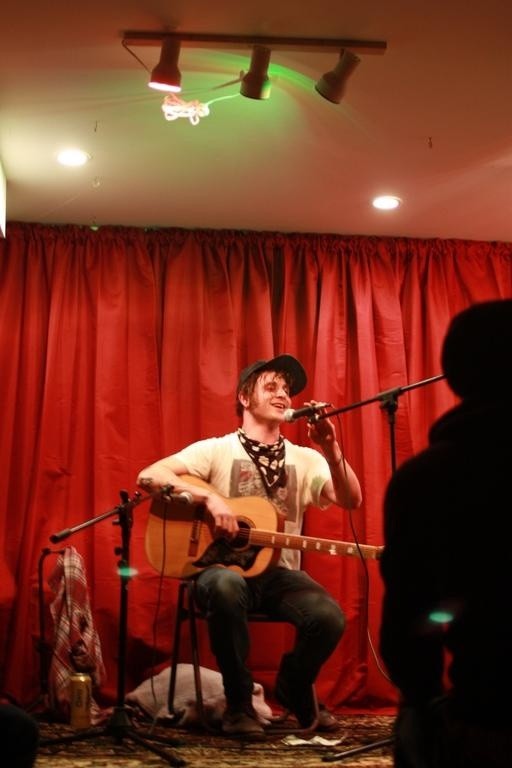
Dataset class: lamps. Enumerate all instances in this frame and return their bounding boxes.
[116,28,388,125]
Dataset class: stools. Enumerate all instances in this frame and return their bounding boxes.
[168,586,321,736]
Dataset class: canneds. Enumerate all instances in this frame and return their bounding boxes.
[69,672,92,732]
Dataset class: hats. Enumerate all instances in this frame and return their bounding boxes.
[237,354,307,399]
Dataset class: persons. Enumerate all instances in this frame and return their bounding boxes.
[134,351,366,743]
[376,296,509,768]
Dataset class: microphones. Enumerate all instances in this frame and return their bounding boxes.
[283,400,330,425]
[160,489,195,507]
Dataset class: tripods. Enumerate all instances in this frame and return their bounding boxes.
[49,497,200,767]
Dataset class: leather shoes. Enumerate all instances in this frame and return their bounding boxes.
[220,706,266,738]
[296,709,341,734]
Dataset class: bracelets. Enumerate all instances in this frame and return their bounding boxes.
[327,454,343,468]
[201,492,214,502]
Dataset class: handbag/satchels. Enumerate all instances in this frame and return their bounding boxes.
[123,662,273,727]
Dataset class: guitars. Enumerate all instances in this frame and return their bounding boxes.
[145,474,385,579]
